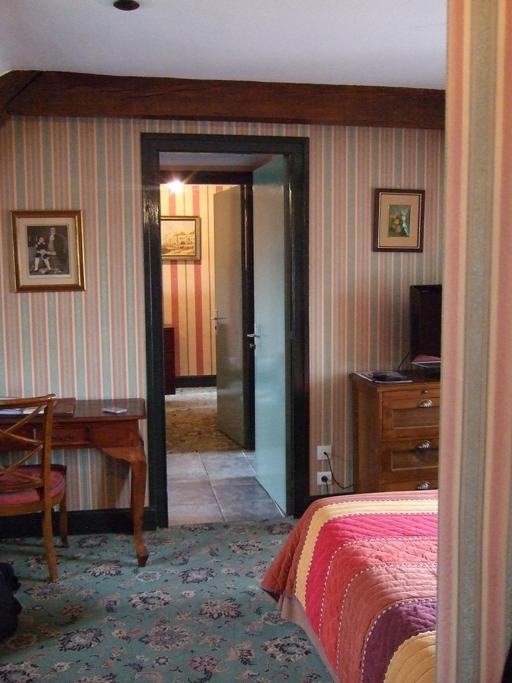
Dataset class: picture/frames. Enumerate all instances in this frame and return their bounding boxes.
[160,214,201,261]
[9,209,88,295]
[372,187,426,253]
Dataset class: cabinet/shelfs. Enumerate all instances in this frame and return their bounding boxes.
[162,323,176,392]
[351,370,441,493]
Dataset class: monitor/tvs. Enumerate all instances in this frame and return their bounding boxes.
[409,284,442,365]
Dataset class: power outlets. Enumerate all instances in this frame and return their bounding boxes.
[315,445,332,461]
[315,470,333,486]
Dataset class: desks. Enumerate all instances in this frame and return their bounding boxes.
[0,396,149,567]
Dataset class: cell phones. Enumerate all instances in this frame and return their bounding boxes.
[102,407,128,414]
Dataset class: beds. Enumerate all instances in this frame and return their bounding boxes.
[262,489,439,683]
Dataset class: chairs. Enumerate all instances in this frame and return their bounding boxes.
[0,392,70,586]
